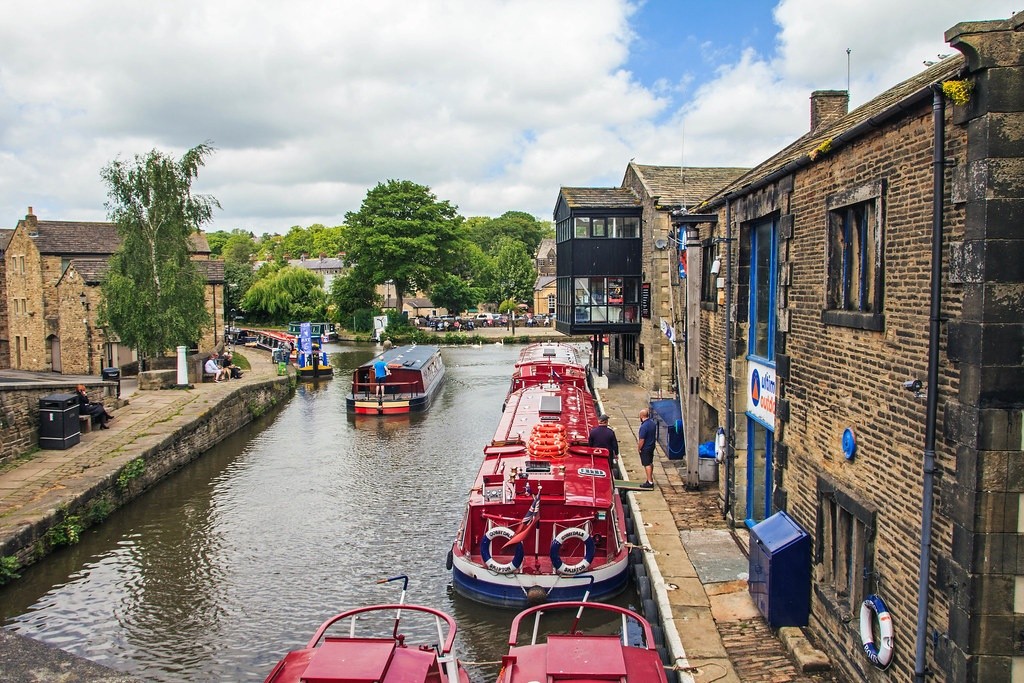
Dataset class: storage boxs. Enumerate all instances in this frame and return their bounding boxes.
[698,458,719,481]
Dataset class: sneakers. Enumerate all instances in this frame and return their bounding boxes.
[640,481,654,488]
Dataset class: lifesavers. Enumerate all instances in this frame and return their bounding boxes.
[527,422,569,457]
[713,428,726,463]
[479,525,524,574]
[859,594,896,671]
[549,527,597,575]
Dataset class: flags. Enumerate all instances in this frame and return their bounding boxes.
[659,319,675,345]
[501,493,539,548]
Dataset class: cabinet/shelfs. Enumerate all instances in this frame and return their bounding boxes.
[648,399,686,460]
[748,510,812,628]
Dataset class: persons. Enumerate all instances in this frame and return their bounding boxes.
[426,315,430,321]
[77,385,114,430]
[589,414,618,467]
[482,317,492,326]
[544,315,550,326]
[468,319,474,329]
[638,410,656,487]
[444,320,449,331]
[205,353,243,382]
[373,355,392,397]
[454,320,459,330]
[528,318,532,326]
[438,322,443,331]
[414,317,419,326]
[383,337,392,351]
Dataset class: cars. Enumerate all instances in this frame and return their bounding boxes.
[410,312,556,327]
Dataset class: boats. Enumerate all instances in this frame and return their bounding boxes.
[225,325,333,379]
[347,380,447,440]
[446,355,629,611]
[288,321,338,340]
[509,340,586,392]
[262,575,470,683]
[346,344,446,415]
[495,575,668,683]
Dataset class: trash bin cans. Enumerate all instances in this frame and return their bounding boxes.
[748,509,813,632]
[101,367,122,398]
[699,457,719,482]
[36,392,81,450]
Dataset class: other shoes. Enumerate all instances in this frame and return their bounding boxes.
[214,380,219,382]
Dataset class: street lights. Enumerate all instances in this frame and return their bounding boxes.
[227,283,238,333]
[385,278,393,310]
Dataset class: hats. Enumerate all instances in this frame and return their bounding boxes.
[598,415,609,422]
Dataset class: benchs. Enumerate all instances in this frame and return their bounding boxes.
[79,407,113,433]
[585,307,622,323]
[204,373,216,383]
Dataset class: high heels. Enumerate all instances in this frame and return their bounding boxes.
[100,424,109,429]
[105,416,115,421]
